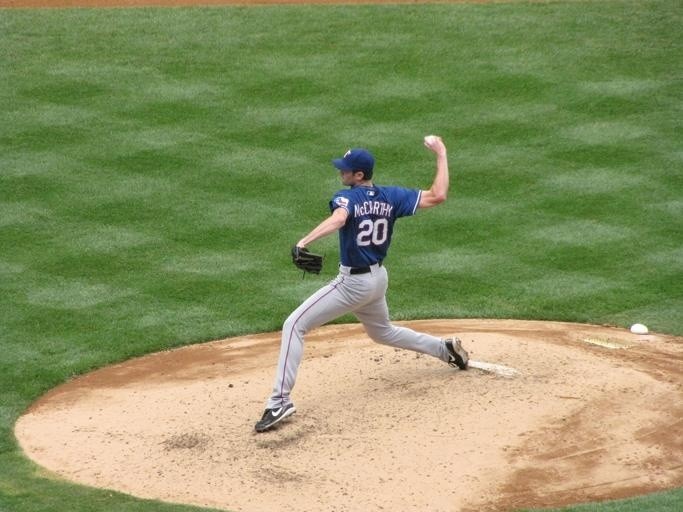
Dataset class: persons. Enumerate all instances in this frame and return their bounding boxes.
[254,134,469,433]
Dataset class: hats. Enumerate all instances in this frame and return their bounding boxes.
[332,149,374,170]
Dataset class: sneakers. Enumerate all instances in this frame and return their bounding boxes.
[443,336,468,370]
[255,403,297,431]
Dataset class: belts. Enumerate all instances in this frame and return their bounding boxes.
[350,262,382,274]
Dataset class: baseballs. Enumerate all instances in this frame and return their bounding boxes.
[426,134,437,143]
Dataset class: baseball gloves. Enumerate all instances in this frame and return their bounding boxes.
[290,243,325,276]
